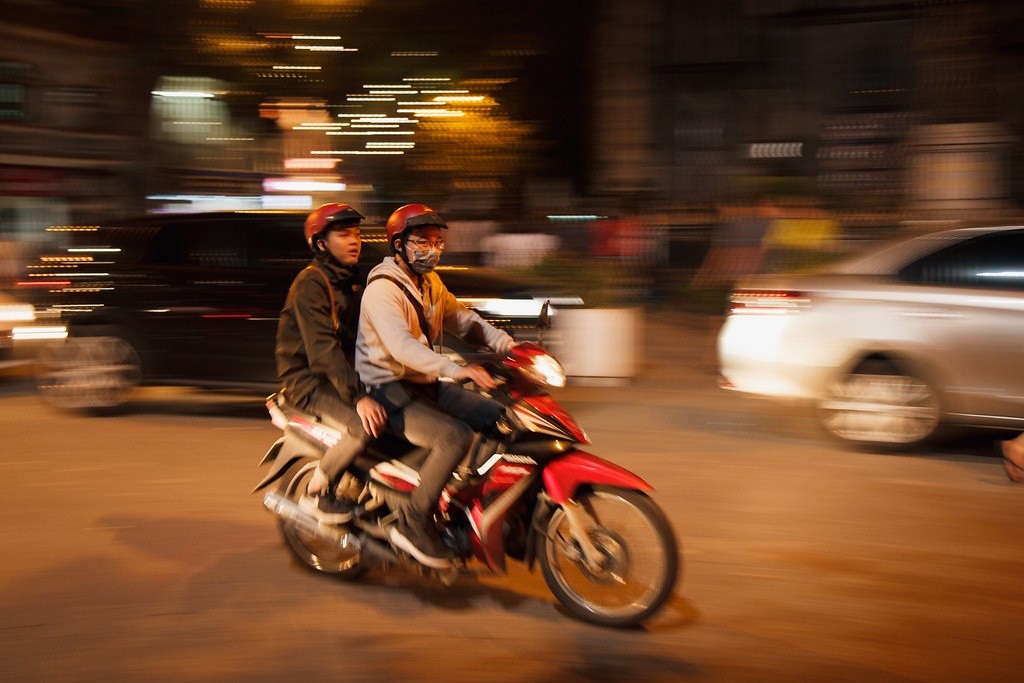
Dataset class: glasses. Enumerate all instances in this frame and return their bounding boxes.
[405,239,447,251]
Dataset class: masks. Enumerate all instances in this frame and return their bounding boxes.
[403,243,442,273]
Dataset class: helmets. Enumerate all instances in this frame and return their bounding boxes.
[304,203,367,253]
[386,203,447,251]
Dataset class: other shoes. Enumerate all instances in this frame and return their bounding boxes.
[298,483,353,525]
[390,523,453,565]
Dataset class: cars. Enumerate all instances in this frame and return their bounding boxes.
[0,192,589,416]
[716,225,1023,454]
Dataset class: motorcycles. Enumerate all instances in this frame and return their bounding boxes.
[247,300,680,628]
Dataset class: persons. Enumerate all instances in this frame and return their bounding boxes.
[354,201,519,570]
[277,202,389,526]
